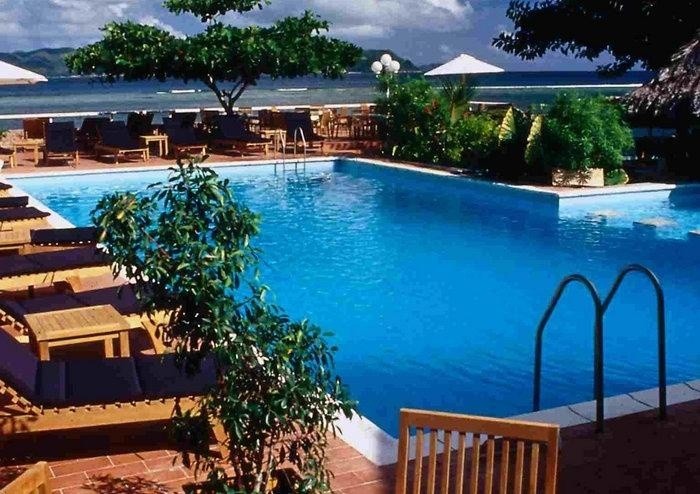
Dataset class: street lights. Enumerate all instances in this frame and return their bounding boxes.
[371,54,401,118]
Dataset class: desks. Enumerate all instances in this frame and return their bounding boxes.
[20,305,134,361]
[13,139,45,167]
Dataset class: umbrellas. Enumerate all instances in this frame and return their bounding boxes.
[422,52,507,86]
[0,59,49,84]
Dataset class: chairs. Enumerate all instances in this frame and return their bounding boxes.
[1,182,120,293]
[23,103,376,168]
[1,277,173,357]
[1,458,53,493]
[1,324,231,462]
[396,408,559,494]
